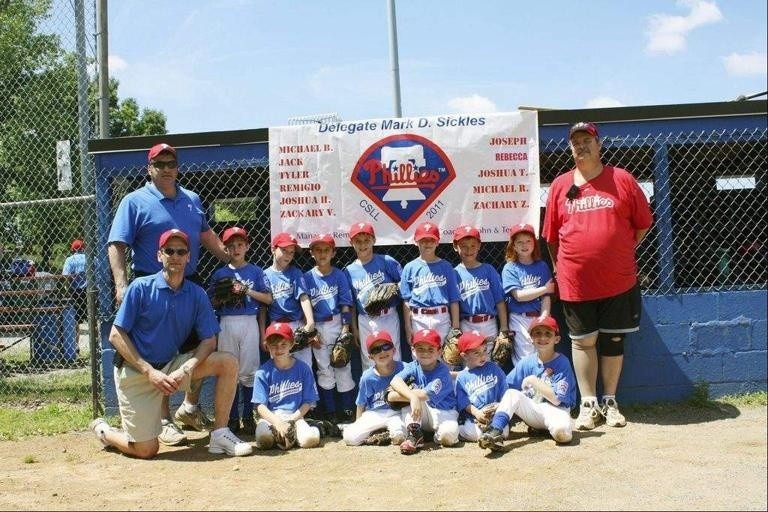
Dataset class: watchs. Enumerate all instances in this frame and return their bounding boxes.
[179,365,192,376]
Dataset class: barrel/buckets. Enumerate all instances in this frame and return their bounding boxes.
[32,298,79,367]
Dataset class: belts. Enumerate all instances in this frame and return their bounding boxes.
[412,305,449,313]
[274,317,296,324]
[319,315,336,322]
[463,314,497,321]
[515,310,541,319]
[371,307,395,316]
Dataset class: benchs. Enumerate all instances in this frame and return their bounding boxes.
[0,268,79,350]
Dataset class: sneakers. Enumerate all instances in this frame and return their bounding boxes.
[477,429,505,452]
[399,423,424,454]
[229,414,256,435]
[575,401,603,430]
[175,401,215,431]
[309,409,354,439]
[601,396,627,427]
[89,418,122,447]
[208,427,252,456]
[157,419,187,446]
[364,432,392,448]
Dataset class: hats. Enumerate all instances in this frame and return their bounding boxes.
[148,143,177,161]
[454,225,480,241]
[271,233,297,248]
[411,328,442,349]
[568,122,599,139]
[457,333,489,351]
[309,233,335,250]
[510,224,535,240]
[222,227,246,244]
[349,222,375,240]
[265,322,293,341]
[414,221,440,242]
[529,317,560,337]
[159,229,190,249]
[71,239,84,250]
[367,331,394,354]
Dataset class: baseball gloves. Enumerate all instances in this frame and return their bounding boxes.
[383,376,415,411]
[210,276,248,313]
[440,328,463,366]
[492,330,516,370]
[268,420,296,450]
[289,327,321,353]
[364,283,402,316]
[363,429,391,447]
[330,331,356,368]
[477,402,523,433]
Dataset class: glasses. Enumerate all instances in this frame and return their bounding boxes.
[369,342,392,354]
[151,160,177,170]
[163,248,188,256]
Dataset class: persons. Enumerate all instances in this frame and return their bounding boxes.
[206,223,579,455]
[108,144,230,447]
[91,229,254,460]
[61,240,90,354]
[545,122,657,431]
